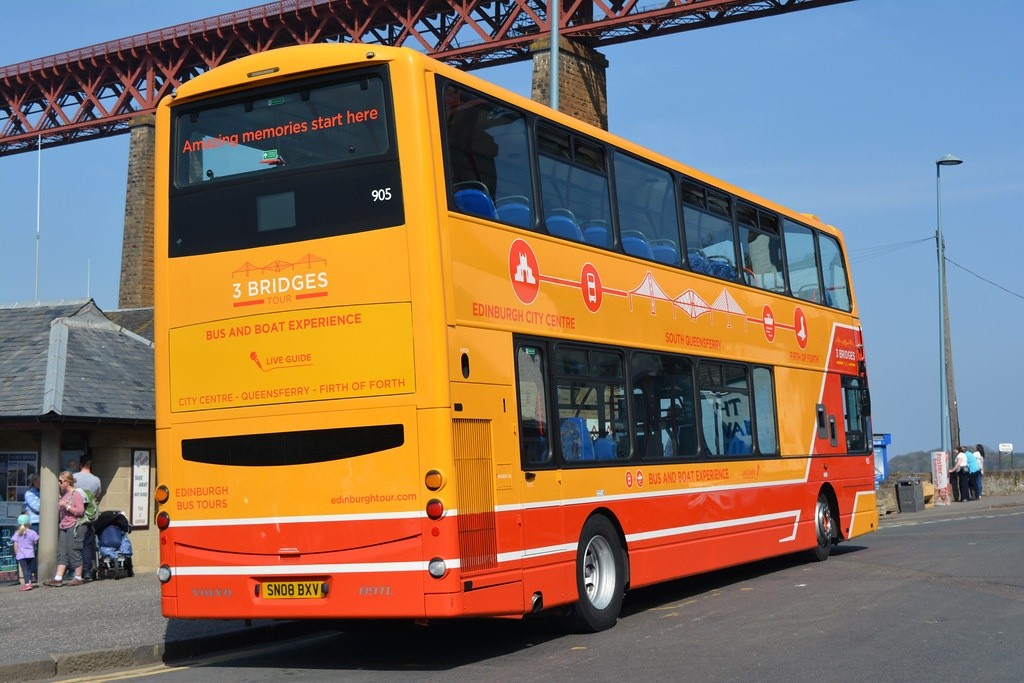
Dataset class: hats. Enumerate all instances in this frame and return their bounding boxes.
[17,515,30,525]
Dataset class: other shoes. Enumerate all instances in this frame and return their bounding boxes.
[66,577,83,586]
[42,578,62,587]
[18,583,33,591]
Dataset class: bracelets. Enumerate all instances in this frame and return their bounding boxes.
[66,504,71,511]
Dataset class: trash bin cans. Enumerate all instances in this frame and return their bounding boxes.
[897,477,925,512]
[916,481,934,508]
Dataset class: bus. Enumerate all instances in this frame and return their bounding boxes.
[153,40,879,633]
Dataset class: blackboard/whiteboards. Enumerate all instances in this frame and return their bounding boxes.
[998,442,1014,453]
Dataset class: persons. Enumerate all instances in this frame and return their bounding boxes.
[664,404,683,455]
[24,454,101,582]
[935,488,948,503]
[948,445,985,502]
[43,471,88,586]
[11,514,40,591]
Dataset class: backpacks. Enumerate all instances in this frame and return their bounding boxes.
[66,488,99,537]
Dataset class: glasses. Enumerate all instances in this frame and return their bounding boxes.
[59,480,66,484]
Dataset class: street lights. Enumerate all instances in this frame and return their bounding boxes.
[933,152,965,451]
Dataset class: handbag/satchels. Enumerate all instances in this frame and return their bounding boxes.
[960,466,970,474]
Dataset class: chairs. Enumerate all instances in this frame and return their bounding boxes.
[525,417,753,466]
[450,181,832,309]
